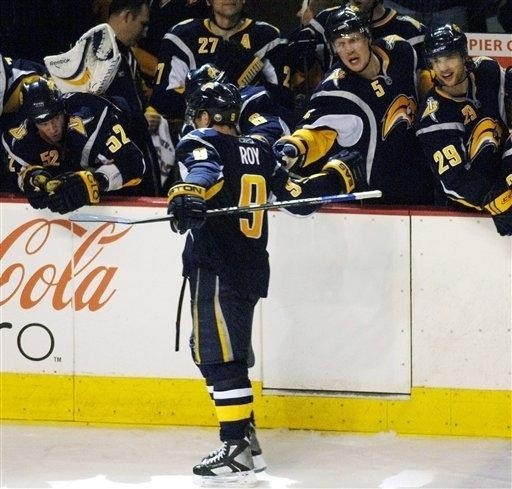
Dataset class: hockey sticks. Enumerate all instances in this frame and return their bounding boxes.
[68,190,382,224]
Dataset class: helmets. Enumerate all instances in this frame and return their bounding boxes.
[424,24,467,58]
[185,81,241,126]
[324,8,370,40]
[18,76,63,123]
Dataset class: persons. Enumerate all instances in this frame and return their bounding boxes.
[0,0,512,235]
[164,81,291,479]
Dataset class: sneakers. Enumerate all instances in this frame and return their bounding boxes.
[193,430,261,475]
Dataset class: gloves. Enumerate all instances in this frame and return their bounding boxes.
[20,165,102,215]
[167,184,207,234]
[322,150,364,194]
[272,139,304,172]
[484,185,512,236]
[186,63,221,94]
